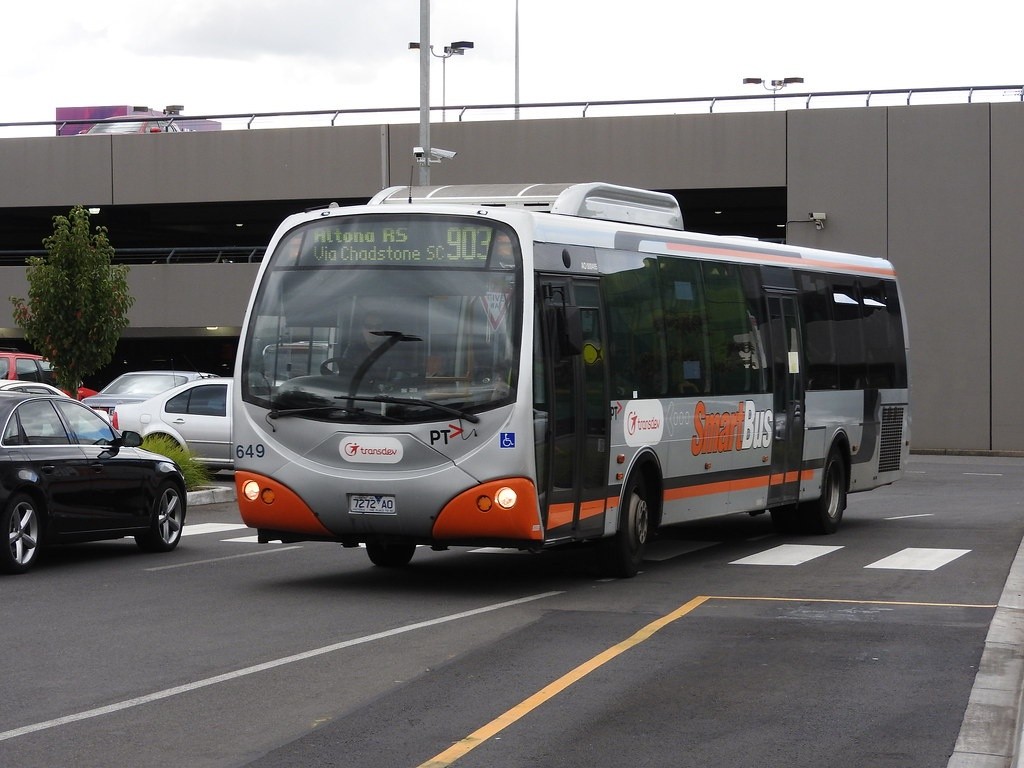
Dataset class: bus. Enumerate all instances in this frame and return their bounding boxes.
[233,182,913,577]
[262,341,340,381]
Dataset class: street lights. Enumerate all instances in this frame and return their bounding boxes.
[743,78,804,112]
[409,41,474,122]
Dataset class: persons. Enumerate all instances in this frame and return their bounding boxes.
[341,310,412,380]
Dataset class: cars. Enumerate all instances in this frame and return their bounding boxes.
[112,377,284,470]
[0,352,98,401]
[0,390,188,574]
[79,371,222,428]
[78,116,181,133]
[0,379,110,439]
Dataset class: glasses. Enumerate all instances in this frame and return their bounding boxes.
[364,323,383,330]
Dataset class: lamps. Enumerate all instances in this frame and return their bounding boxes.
[163,105,184,115]
[743,77,804,93]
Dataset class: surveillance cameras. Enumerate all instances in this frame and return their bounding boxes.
[430,148,457,160]
[413,147,424,160]
[809,212,826,220]
